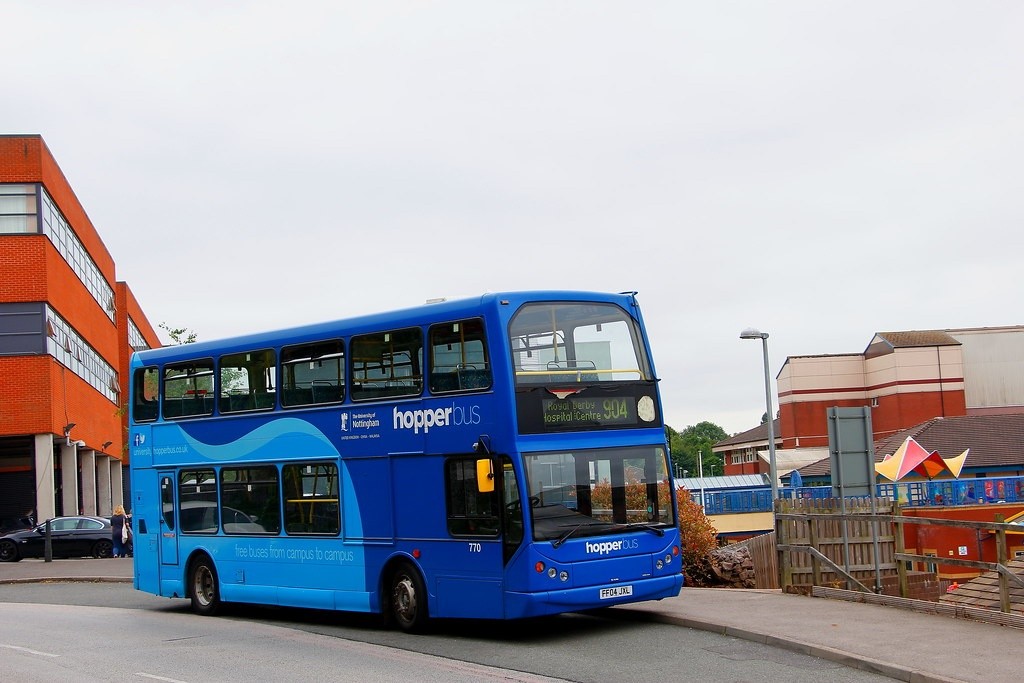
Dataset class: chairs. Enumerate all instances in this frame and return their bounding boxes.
[286,523,308,532]
[182,360,598,416]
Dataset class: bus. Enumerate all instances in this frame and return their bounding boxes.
[127,292,684,636]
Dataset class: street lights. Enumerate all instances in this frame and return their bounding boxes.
[741,329,778,533]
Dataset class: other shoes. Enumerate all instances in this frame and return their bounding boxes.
[114,554,118,558]
[125,554,128,558]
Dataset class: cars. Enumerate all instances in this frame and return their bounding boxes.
[1,517,132,562]
[161,502,266,534]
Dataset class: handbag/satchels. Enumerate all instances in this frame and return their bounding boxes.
[122,518,128,544]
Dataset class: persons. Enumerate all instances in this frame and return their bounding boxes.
[111,505,132,557]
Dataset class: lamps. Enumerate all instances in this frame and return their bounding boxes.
[63,423,76,434]
[102,442,112,450]
